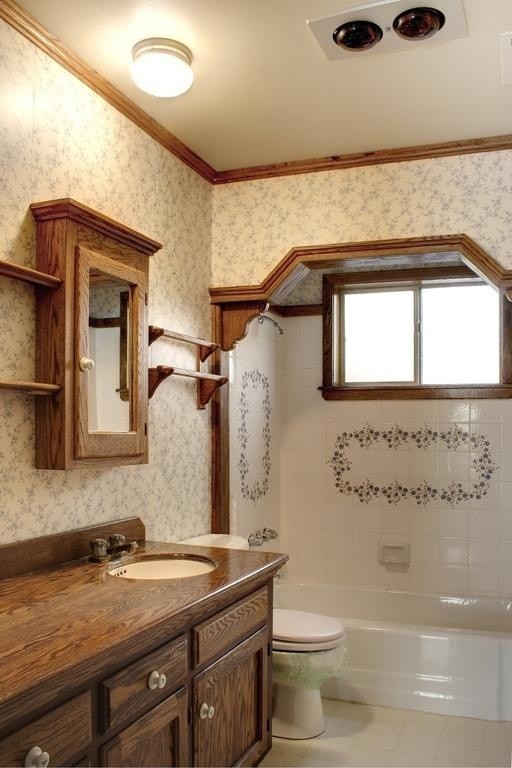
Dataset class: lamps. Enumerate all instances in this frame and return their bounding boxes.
[129,38,194,97]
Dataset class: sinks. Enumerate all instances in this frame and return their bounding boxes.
[108,552,218,580]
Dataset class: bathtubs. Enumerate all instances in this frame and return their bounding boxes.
[272,581,512,723]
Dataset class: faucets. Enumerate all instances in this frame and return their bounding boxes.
[259,532,270,544]
[106,541,138,555]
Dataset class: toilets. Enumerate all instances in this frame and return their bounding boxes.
[177,534,345,740]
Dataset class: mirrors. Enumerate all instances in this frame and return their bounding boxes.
[75,246,147,458]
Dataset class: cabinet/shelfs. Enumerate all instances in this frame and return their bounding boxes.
[29,198,163,470]
[148,326,228,409]
[0,259,62,399]
[0,559,274,768]
